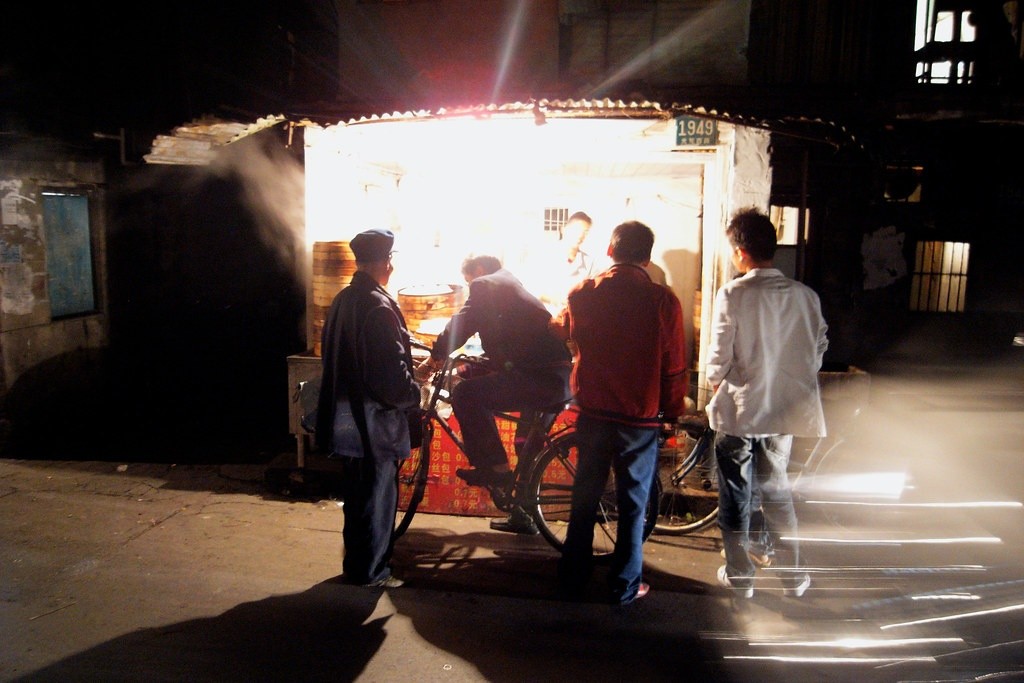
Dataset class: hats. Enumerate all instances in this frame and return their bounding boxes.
[349,229,394,261]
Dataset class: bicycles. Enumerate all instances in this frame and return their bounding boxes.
[616,413,723,537]
[390,339,663,565]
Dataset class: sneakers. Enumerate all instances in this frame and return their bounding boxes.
[783,573,811,597]
[456,467,514,485]
[716,564,754,601]
[489,515,542,538]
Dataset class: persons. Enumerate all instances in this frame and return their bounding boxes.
[529,211,596,320]
[420,249,573,535]
[316,228,420,589]
[703,206,828,598]
[549,220,688,608]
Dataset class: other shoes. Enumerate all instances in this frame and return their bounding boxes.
[359,573,405,588]
[617,581,651,604]
